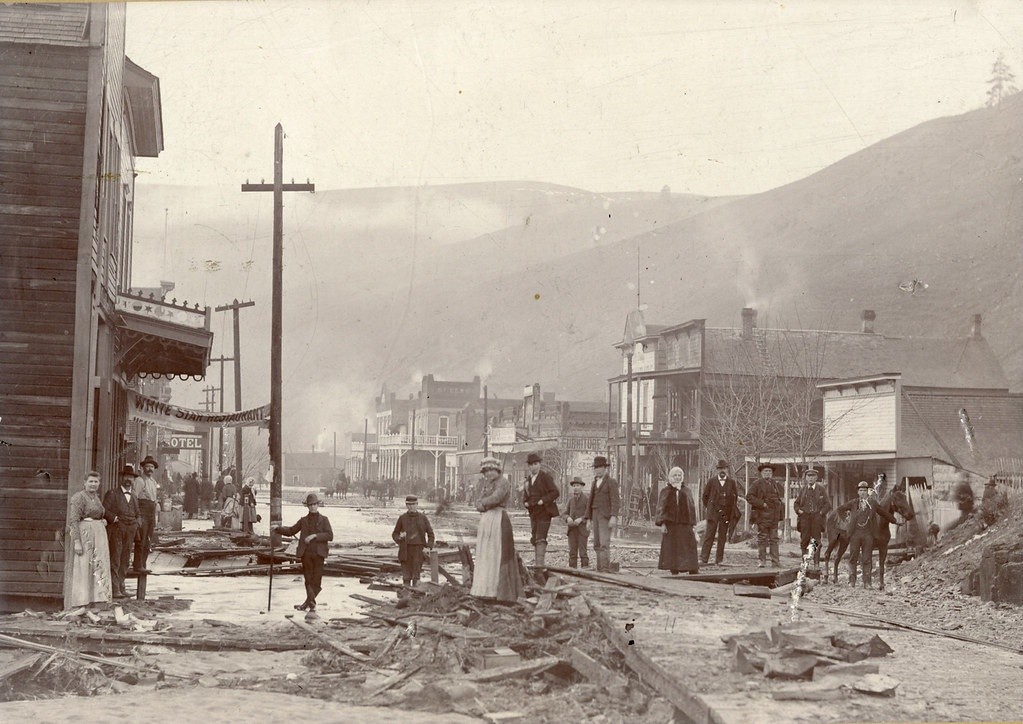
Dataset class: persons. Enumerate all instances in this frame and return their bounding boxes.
[982,480,998,502]
[560,477,589,569]
[699,459,738,566]
[133,456,159,574]
[103,466,141,598]
[470,457,526,604]
[794,469,832,571]
[162,469,257,533]
[523,453,559,566]
[70,471,113,607]
[392,496,434,586]
[955,473,974,517]
[270,494,333,612]
[837,481,904,589]
[655,467,699,575]
[337,468,474,506]
[746,462,785,568]
[585,456,621,573]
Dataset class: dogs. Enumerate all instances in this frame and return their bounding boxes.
[325,490,335,499]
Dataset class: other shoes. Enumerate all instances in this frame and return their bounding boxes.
[134,566,153,574]
[697,559,707,564]
[848,581,856,588]
[863,583,873,590]
[114,590,136,598]
[714,561,724,566]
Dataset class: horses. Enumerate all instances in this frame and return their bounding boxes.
[425,487,445,502]
[363,478,393,500]
[335,476,350,499]
[823,480,914,590]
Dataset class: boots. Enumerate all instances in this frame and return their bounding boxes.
[403,579,421,587]
[294,585,321,612]
[758,545,766,568]
[533,541,547,566]
[770,544,787,569]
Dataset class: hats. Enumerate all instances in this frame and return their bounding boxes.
[140,455,159,468]
[527,454,543,463]
[570,476,585,486]
[757,462,777,472]
[856,481,872,488]
[592,457,611,467]
[805,468,819,476]
[716,459,730,468]
[118,465,139,478]
[984,480,997,487]
[479,457,503,473]
[303,494,320,505]
[405,494,419,503]
